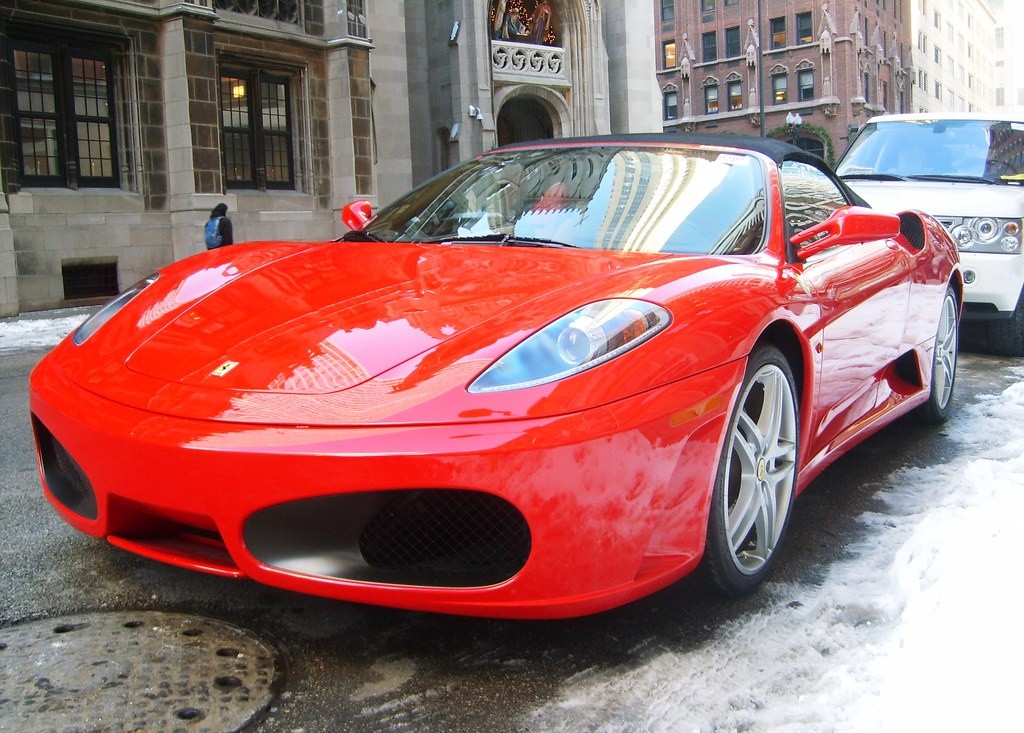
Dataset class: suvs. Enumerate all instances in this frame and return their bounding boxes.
[830,111,1024,358]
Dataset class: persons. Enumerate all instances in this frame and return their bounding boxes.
[204,202,233,251]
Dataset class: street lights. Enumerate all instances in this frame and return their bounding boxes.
[785,112,802,147]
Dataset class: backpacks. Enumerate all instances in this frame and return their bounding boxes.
[204,216,222,248]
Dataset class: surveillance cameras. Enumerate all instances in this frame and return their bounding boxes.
[477,114,483,121]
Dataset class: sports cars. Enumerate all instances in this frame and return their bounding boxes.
[26,133,965,609]
